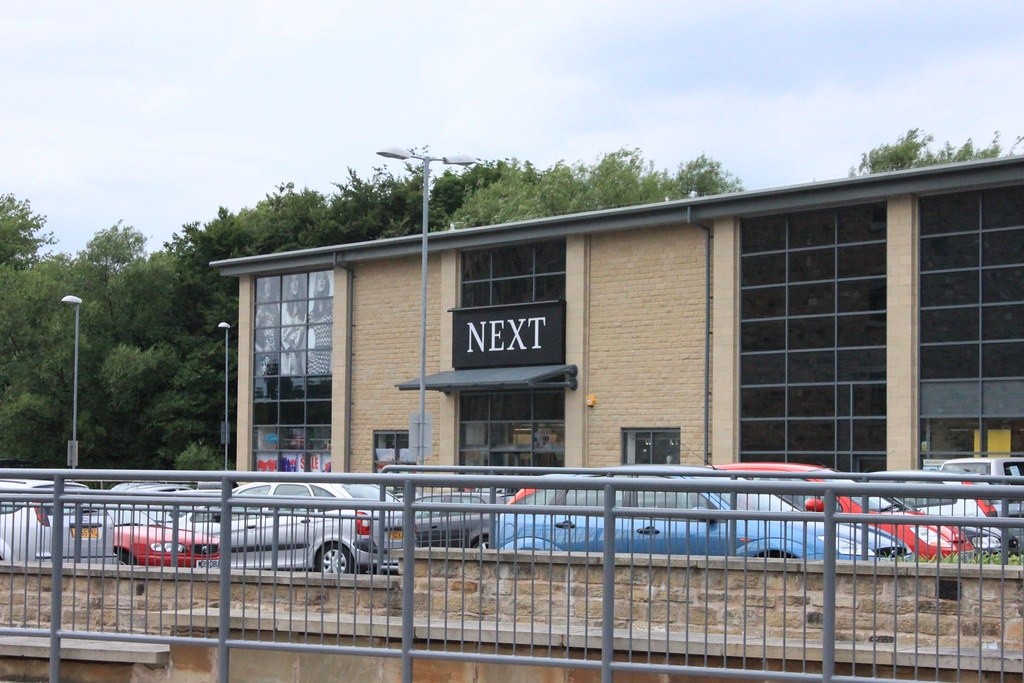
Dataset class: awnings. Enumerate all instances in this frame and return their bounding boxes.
[392,364,574,390]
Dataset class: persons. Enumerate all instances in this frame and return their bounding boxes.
[281,273,309,376]
[254,277,280,376]
[295,270,334,376]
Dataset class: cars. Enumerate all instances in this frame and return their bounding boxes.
[867,470,998,518]
[180,482,418,573]
[0,479,118,564]
[931,514,1020,557]
[109,482,159,491]
[414,493,516,549]
[940,458,1024,514]
[124,483,192,492]
[108,509,220,568]
[706,462,977,558]
[495,463,914,563]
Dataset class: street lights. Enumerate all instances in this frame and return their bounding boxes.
[218,321,230,471]
[61,294,83,469]
[377,147,476,465]
